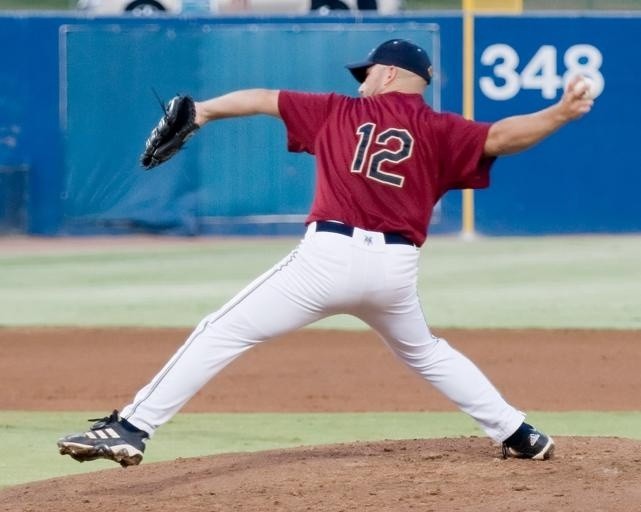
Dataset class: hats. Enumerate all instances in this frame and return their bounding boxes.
[345,39,433,83]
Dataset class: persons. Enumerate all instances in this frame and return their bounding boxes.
[55,36,597,466]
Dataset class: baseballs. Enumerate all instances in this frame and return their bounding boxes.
[573,76,598,100]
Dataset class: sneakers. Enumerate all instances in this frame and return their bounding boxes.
[58,410,150,465]
[502,426,556,460]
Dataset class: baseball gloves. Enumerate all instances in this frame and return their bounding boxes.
[140,95,200,171]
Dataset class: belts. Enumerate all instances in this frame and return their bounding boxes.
[317,220,421,246]
